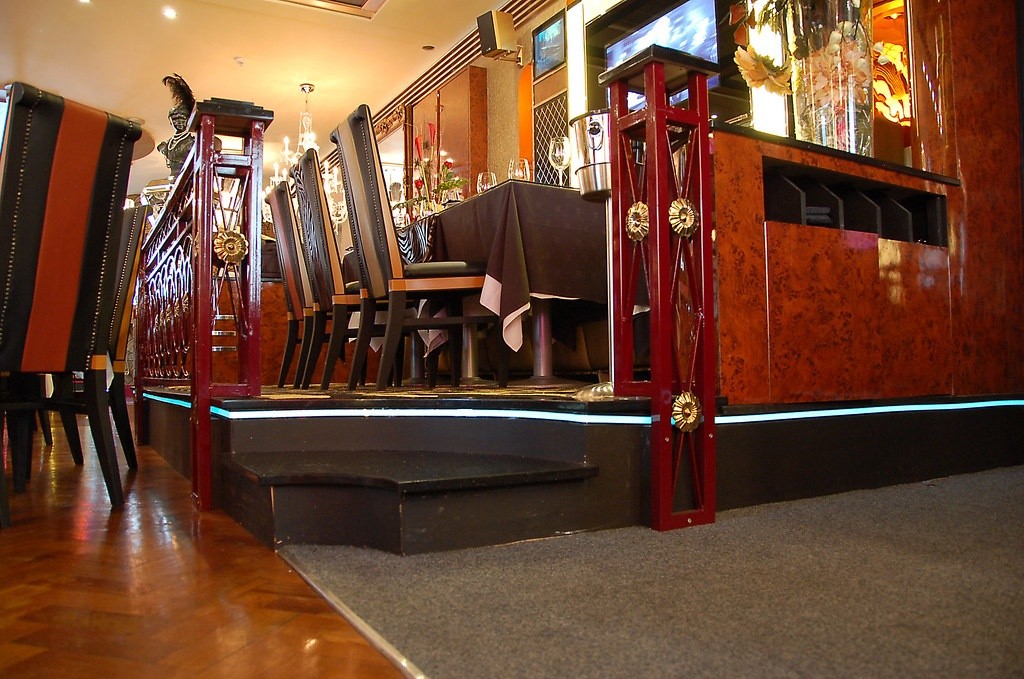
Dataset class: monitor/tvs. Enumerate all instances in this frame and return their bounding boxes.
[604,0,722,109]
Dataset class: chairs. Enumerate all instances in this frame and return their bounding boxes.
[264,104,506,390]
[1,78,151,509]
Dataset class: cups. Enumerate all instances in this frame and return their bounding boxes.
[475,172,498,194]
[442,188,464,208]
[507,158,531,181]
[421,200,437,217]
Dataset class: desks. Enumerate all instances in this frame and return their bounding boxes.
[343,179,608,388]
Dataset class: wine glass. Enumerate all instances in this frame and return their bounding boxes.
[548,137,572,186]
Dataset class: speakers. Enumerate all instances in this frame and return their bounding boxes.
[477,10,518,59]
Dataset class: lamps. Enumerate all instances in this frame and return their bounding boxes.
[271,83,349,236]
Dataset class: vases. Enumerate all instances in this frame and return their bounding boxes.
[441,187,464,208]
[420,199,437,218]
[786,0,874,159]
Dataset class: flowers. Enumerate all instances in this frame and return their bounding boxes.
[414,180,424,196]
[415,122,436,200]
[728,0,872,103]
[432,160,470,193]
